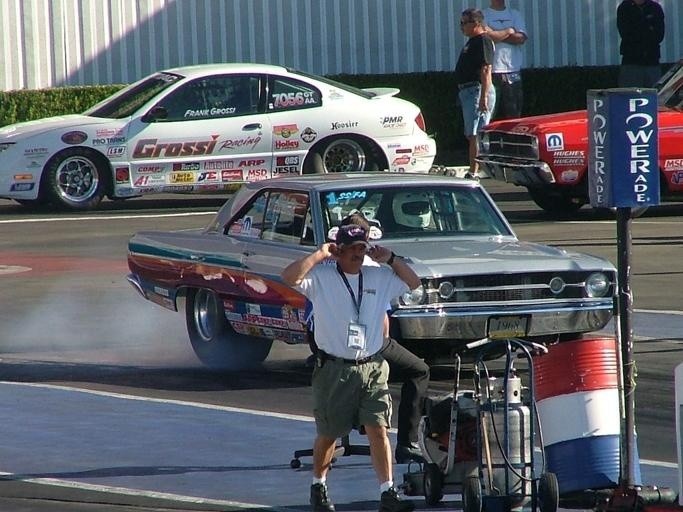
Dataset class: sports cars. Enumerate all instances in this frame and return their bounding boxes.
[475,60,683,210]
[127,171,617,367]
[1,62,438,204]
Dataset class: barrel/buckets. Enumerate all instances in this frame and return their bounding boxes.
[532,338,642,492]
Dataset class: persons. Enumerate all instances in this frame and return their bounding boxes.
[617,0,665,89]
[305,215,432,463]
[455,7,497,182]
[478,0,528,119]
[281,225,426,512]
[391,202,438,232]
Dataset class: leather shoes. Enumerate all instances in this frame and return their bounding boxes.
[395,443,426,463]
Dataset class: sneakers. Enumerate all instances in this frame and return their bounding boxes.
[464,173,479,182]
[379,487,414,512]
[310,483,335,512]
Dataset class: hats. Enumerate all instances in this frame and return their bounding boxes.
[336,224,370,250]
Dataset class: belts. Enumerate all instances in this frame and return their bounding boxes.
[327,355,369,364]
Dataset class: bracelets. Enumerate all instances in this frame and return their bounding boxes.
[387,249,395,266]
